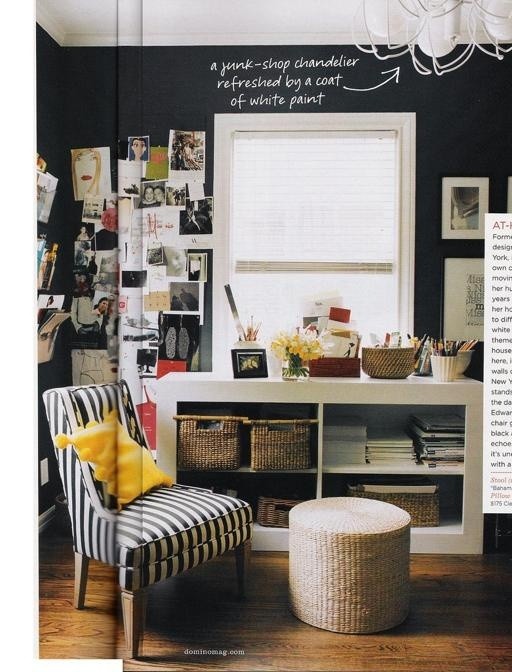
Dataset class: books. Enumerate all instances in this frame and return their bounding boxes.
[363,405,467,471]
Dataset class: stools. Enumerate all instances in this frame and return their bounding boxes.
[287,495,412,635]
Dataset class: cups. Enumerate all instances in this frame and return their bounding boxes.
[430,355,458,382]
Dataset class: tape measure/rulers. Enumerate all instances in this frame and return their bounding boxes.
[224,284,246,340]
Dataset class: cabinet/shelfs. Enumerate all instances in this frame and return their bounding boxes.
[156,371,484,555]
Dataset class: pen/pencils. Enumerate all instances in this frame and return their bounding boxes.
[431,336,458,356]
[407,333,431,375]
[244,315,261,341]
[458,337,479,350]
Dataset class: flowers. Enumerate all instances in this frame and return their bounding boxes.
[271,331,325,376]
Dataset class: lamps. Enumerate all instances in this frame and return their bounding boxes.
[349,0,512,76]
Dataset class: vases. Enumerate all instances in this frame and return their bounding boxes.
[281,353,310,383]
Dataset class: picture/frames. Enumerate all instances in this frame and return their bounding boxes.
[231,348,268,378]
[441,177,489,239]
[438,253,485,349]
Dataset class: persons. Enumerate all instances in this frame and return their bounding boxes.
[36,126,208,367]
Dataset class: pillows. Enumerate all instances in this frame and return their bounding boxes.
[56,410,174,514]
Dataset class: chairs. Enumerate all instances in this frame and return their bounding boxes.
[41,375,253,658]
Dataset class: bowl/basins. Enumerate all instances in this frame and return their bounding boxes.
[457,348,476,380]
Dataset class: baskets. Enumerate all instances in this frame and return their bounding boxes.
[347,484,440,527]
[362,347,414,378]
[251,423,311,470]
[178,420,239,471]
[257,497,305,527]
